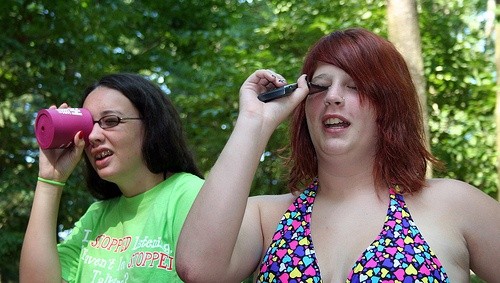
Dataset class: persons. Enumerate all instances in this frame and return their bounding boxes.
[18,73,249,283]
[175,27,499,283]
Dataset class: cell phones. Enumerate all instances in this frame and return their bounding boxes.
[257,82,299,102]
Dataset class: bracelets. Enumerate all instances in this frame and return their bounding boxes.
[37,177,66,187]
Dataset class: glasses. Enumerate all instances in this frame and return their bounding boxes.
[93,115,142,129]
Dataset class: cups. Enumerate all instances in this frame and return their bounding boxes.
[35,107,94,151]
